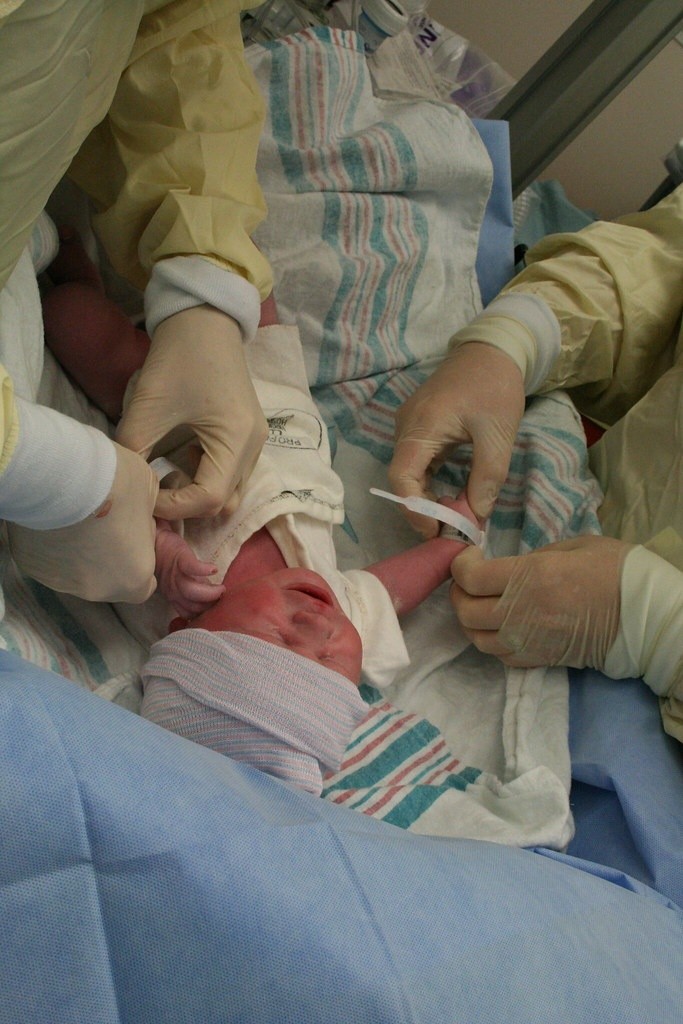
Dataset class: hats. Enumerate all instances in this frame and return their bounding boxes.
[140,628,369,798]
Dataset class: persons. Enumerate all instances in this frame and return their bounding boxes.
[32,262,490,800]
[0,0,275,605]
[384,184,683,744]
